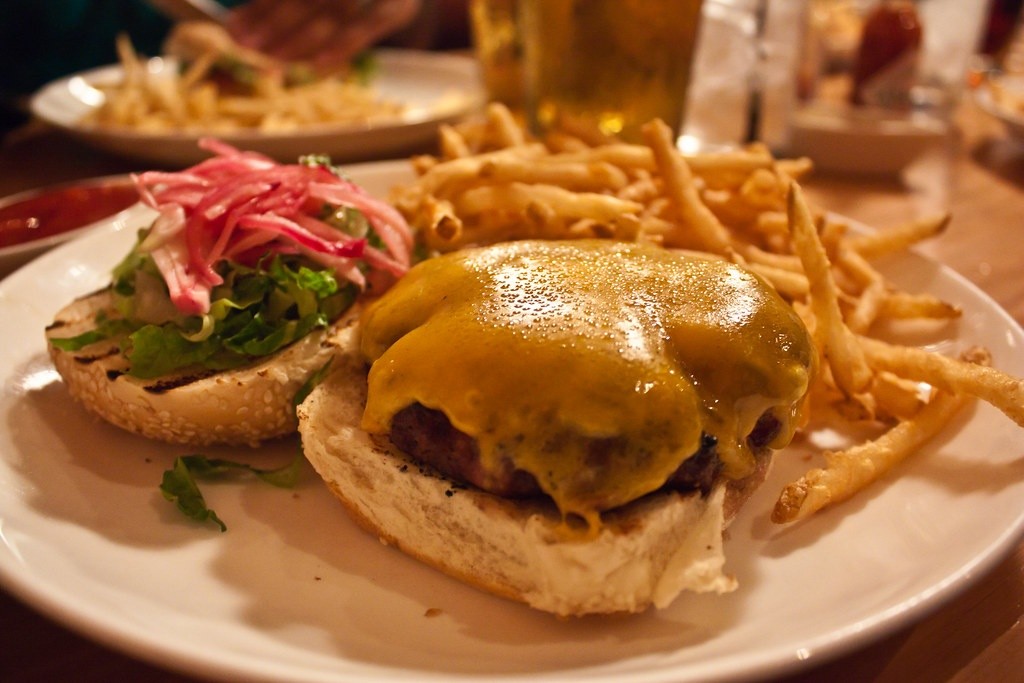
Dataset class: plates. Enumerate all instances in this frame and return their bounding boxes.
[0,155,1022,679]
[28,52,481,161]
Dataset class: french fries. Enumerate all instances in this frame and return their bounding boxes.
[384,103,1023,526]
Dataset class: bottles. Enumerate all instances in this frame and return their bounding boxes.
[848,0,924,109]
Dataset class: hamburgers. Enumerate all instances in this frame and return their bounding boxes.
[46,137,814,620]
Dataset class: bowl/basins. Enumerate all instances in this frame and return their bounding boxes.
[791,114,938,174]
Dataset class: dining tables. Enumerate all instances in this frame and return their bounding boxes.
[3,125,1024,683]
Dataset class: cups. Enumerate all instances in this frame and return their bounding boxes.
[470,0,701,148]
